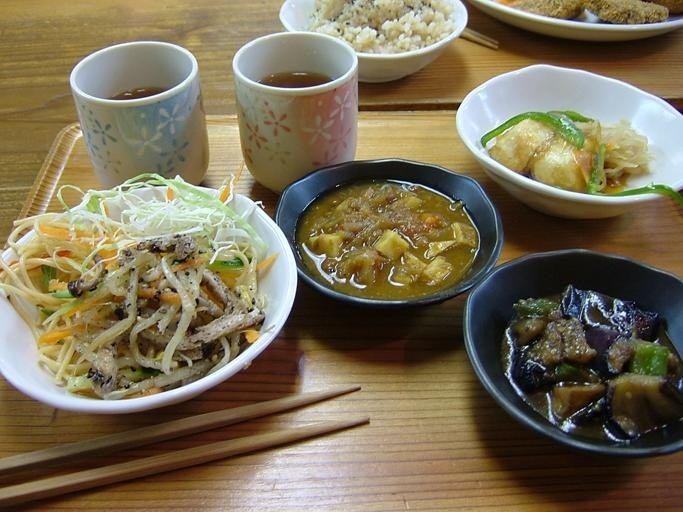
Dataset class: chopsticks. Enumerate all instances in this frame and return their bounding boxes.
[460,27,500,52]
[1,383,370,512]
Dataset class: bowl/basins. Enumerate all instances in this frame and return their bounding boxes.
[273,158,505,312]
[0,185,298,415]
[463,248,683,459]
[455,63,683,221]
[279,0,469,83]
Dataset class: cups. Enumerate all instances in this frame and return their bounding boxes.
[232,30,359,193]
[69,40,211,191]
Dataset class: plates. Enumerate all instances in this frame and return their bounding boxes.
[469,1,683,43]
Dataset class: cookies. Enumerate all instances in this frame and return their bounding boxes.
[518,0,584,19]
[647,0,682,14]
[585,1,668,23]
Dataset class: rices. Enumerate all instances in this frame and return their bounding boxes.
[307,0,440,55]
